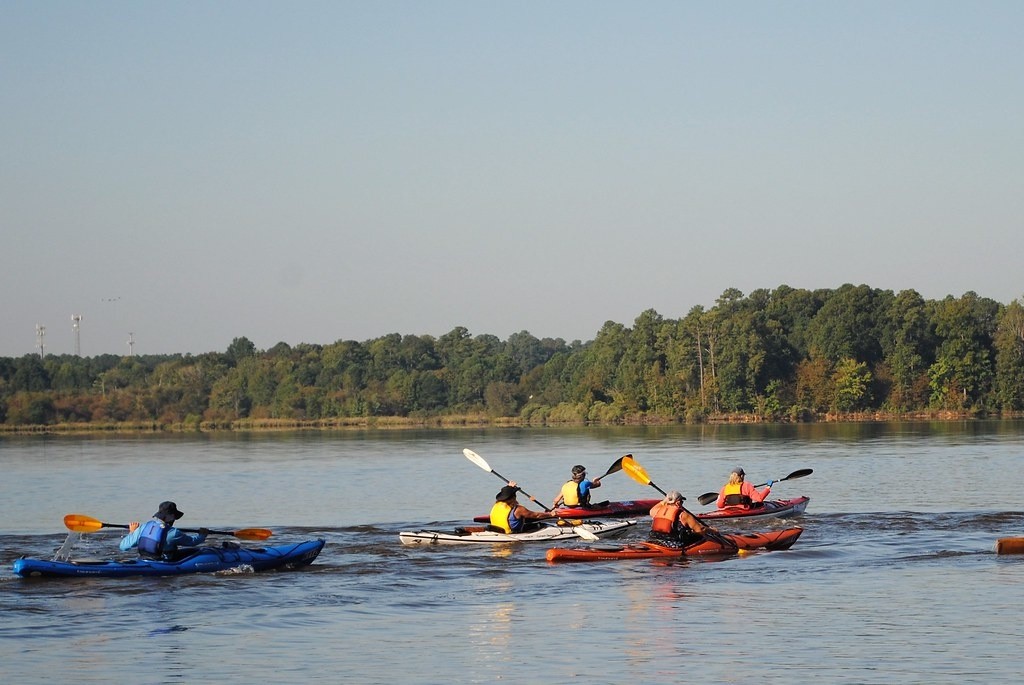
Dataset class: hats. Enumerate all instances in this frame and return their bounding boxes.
[495,485,520,500]
[667,489,686,504]
[152,501,184,522]
[576,469,588,475]
[732,467,746,476]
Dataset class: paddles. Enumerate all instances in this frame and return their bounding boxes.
[550,453,633,512]
[696,468,814,506]
[622,457,748,553]
[64,514,272,540]
[463,448,600,540]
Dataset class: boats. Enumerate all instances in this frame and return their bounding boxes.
[15,537,327,578]
[693,494,813,521]
[546,525,806,565]
[399,519,639,547]
[473,499,668,524]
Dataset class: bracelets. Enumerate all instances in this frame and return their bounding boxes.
[660,501,664,506]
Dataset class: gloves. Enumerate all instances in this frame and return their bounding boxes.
[198,527,209,537]
[767,479,773,487]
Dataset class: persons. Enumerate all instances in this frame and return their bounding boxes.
[716,467,772,510]
[650,491,718,548]
[119,501,210,562]
[552,465,601,509]
[490,481,558,534]
